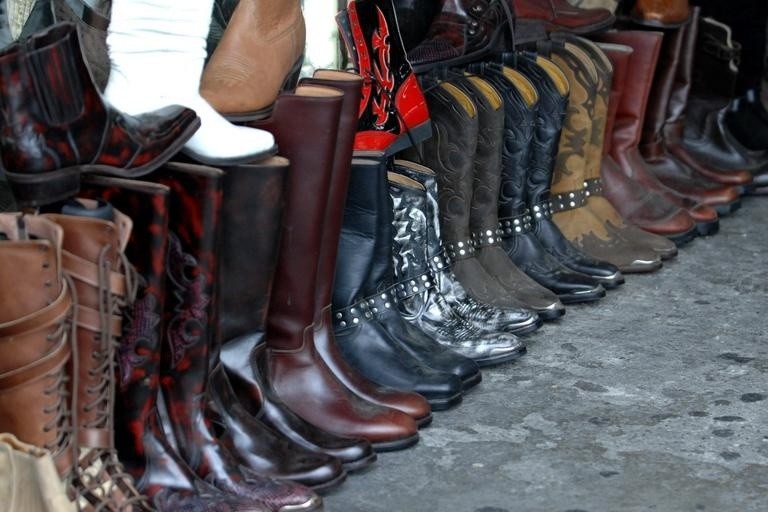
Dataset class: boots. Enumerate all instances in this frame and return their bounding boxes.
[1,1,768,511]
